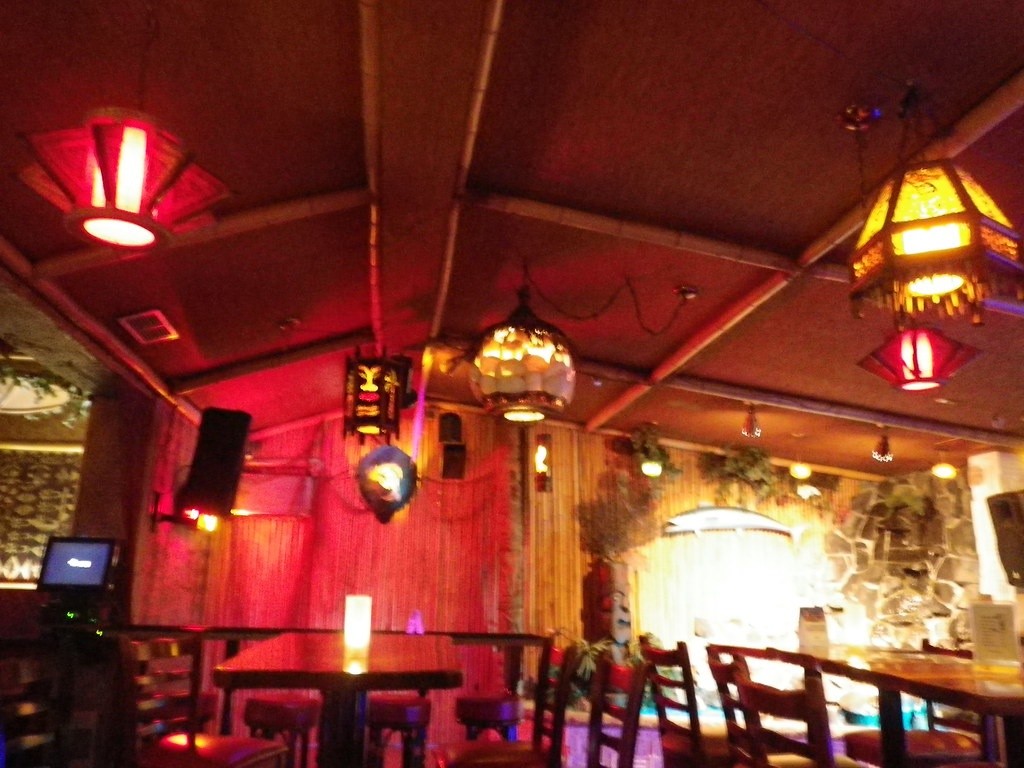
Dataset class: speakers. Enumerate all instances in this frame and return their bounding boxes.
[184,406,253,514]
[987,490,1023,587]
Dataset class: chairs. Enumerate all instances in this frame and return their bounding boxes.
[0,632,72,768]
[460,639,577,768]
[108,625,292,768]
[636,635,989,768]
[581,648,655,768]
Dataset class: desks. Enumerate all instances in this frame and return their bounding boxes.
[710,627,1024,767]
[214,631,463,768]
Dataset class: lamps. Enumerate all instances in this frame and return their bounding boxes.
[3,104,231,246]
[462,281,577,429]
[641,397,958,501]
[843,73,1024,330]
[149,346,423,540]
[857,311,982,395]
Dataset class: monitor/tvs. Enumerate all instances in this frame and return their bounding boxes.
[36,536,122,593]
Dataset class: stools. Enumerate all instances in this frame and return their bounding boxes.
[454,692,523,746]
[237,690,323,767]
[363,690,432,768]
